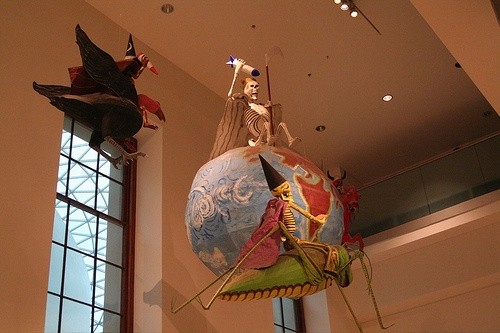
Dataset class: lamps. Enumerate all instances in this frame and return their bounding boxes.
[333,0,343,4]
[339,3,351,11]
[350,8,358,18]
